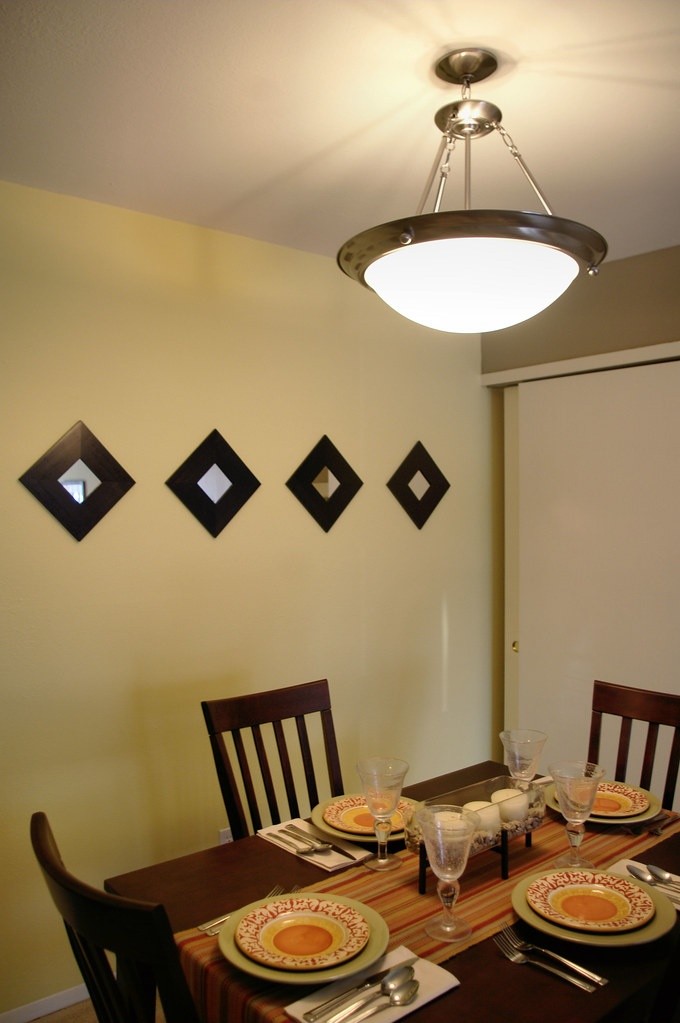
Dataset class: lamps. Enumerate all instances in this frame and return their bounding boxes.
[336,45,608,333]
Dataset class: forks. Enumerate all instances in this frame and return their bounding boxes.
[205,885,302,936]
[197,884,285,931]
[492,934,596,994]
[497,920,609,987]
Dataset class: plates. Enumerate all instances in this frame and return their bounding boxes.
[544,779,663,824]
[234,897,371,970]
[510,867,677,947]
[526,871,656,934]
[311,792,425,842]
[552,782,650,817]
[322,795,416,835]
[218,892,390,984]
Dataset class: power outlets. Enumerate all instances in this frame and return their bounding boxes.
[218,828,233,843]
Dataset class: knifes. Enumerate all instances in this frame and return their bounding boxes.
[285,824,356,860]
[303,957,420,1023]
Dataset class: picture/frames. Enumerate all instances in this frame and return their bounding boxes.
[164,429,262,539]
[18,420,136,542]
[386,441,451,530]
[286,434,364,534]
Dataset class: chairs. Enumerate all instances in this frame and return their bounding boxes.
[199,679,344,842]
[30,811,197,1023]
[585,679,680,811]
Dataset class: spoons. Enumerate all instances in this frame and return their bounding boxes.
[278,829,332,852]
[646,865,680,887]
[322,965,415,1023]
[626,864,680,894]
[266,832,315,854]
[345,979,420,1023]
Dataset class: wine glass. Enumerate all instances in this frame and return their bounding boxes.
[415,804,481,943]
[499,728,549,782]
[548,760,606,871]
[356,757,410,872]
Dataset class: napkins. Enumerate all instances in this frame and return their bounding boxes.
[284,945,460,1023]
[257,818,374,872]
[607,858,680,911]
[533,775,573,788]
